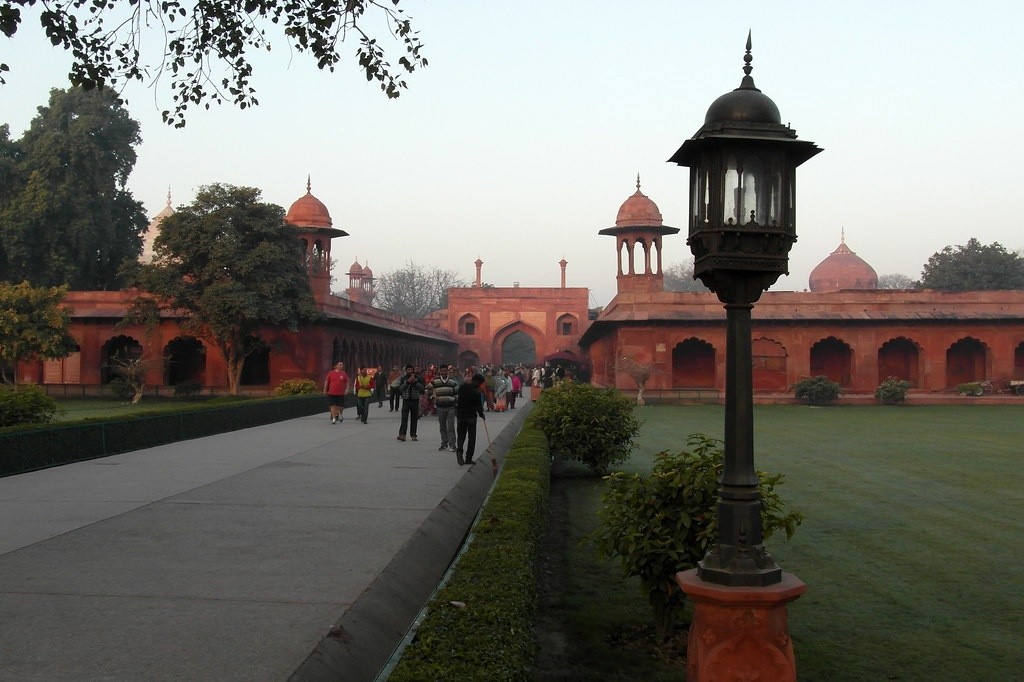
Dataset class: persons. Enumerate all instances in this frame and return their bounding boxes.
[456,374,486,466]
[322,362,349,425]
[402,361,564,417]
[387,365,403,412]
[397,365,425,442]
[484,371,495,412]
[424,364,460,452]
[373,365,388,408]
[354,366,375,424]
[510,372,522,409]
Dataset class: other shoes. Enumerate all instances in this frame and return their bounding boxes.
[397,433,405,441]
[464,460,476,464]
[439,445,448,451]
[449,446,456,452]
[332,418,336,424]
[361,419,368,424]
[340,415,343,423]
[456,451,464,465]
[355,417,362,420]
[411,434,417,441]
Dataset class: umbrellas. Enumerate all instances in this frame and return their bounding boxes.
[542,349,578,364]
